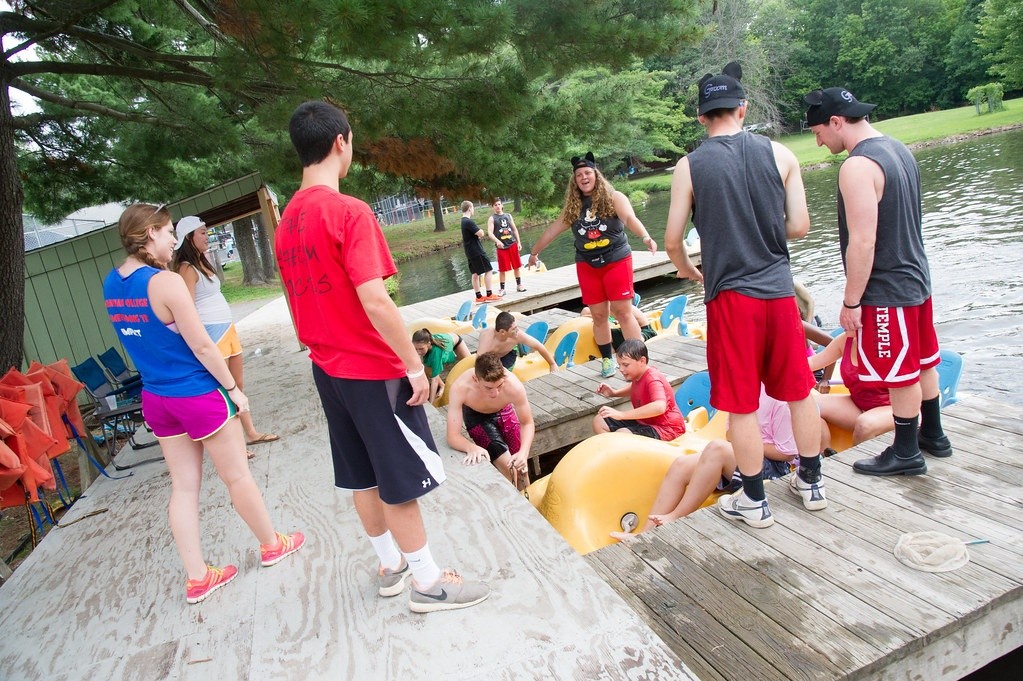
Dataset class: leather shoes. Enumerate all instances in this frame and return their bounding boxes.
[916,427,952,457]
[853,445,927,476]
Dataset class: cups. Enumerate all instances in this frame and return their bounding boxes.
[105,395,118,411]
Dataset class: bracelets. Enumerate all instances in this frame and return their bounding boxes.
[642,236,647,241]
[531,253,537,256]
[843,300,861,309]
[226,382,237,391]
[405,363,425,378]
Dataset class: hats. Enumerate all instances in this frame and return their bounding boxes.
[173,216,205,252]
[697,62,745,115]
[803,86,877,127]
[571,151,595,172]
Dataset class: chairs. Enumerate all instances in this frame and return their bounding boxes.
[672,372,717,421]
[97,347,159,445]
[70,357,164,470]
[936,351,963,407]
[459,294,691,371]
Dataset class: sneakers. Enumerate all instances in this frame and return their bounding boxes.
[376,552,412,597]
[408,570,491,611]
[486,295,501,300]
[185,565,238,603]
[789,471,827,511]
[718,487,774,528]
[476,296,493,304]
[498,289,506,296]
[260,532,305,566]
[517,287,526,292]
[602,358,616,378]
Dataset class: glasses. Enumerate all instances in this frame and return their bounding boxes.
[145,202,167,223]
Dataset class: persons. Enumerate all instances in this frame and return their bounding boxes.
[804,86,952,476]
[478,312,559,374]
[663,74,827,528]
[806,330,896,457]
[592,338,686,442]
[224,238,233,258]
[528,150,658,378]
[460,201,503,304]
[275,100,491,613]
[412,328,472,404]
[174,215,280,459]
[609,381,819,542]
[446,351,535,490]
[792,278,837,395]
[487,196,527,296]
[103,204,305,603]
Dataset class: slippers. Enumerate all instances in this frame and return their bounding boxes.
[246,433,279,445]
